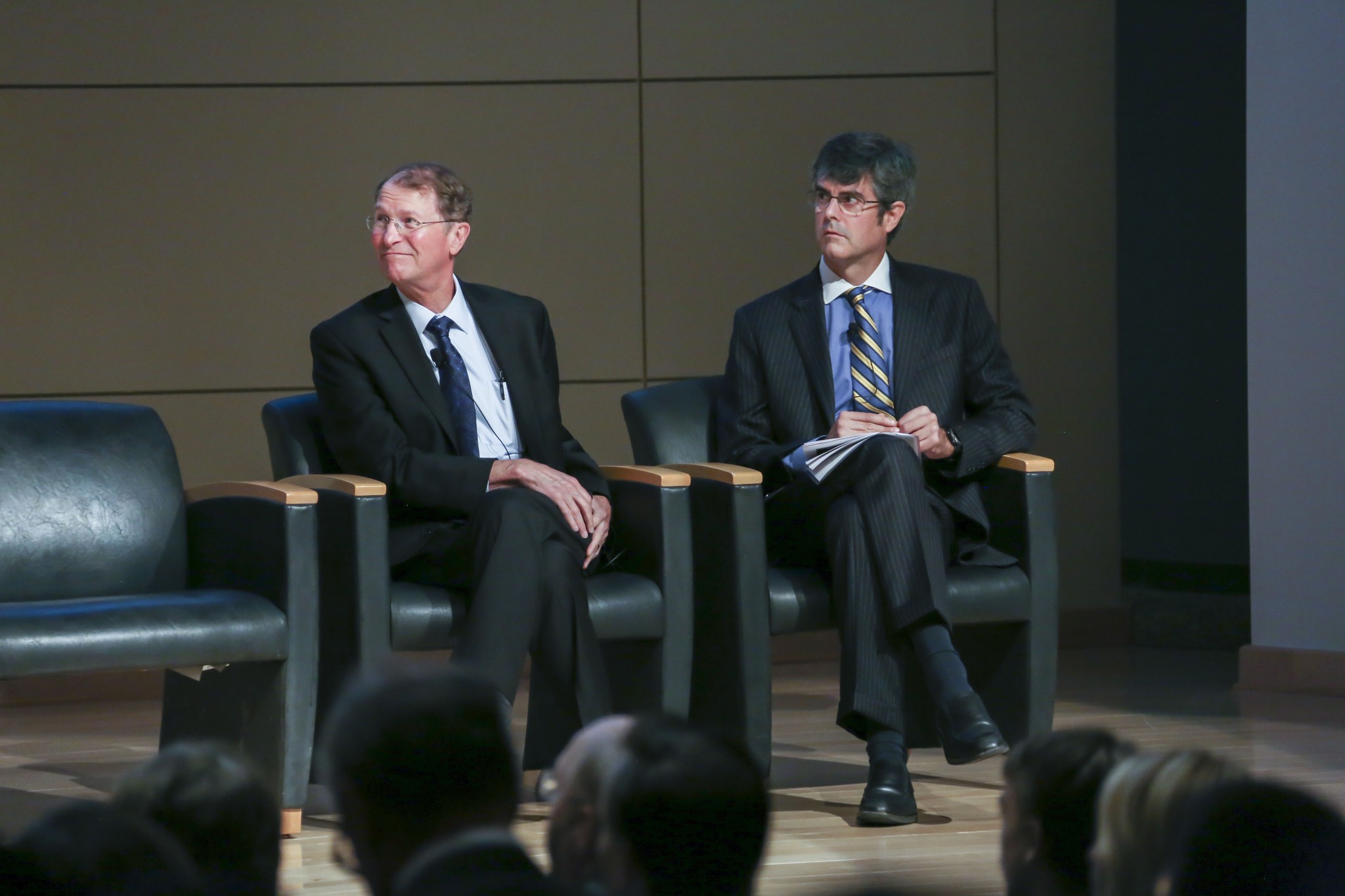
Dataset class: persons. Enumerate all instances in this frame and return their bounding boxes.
[612,714,772,896]
[0,798,213,896]
[325,665,570,896]
[998,729,1137,896]
[1153,781,1345,896]
[310,163,613,740]
[722,132,1037,827]
[123,740,280,896]
[542,710,641,896]
[1090,750,1252,896]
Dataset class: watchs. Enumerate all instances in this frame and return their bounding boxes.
[944,427,962,451]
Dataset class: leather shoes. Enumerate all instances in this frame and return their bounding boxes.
[856,763,918,823]
[936,694,1008,766]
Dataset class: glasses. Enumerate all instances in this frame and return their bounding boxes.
[807,191,885,214]
[365,214,456,235]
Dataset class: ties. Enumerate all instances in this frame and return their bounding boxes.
[425,315,479,459]
[842,285,896,420]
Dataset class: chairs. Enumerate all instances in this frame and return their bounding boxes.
[260,391,693,812]
[619,373,1061,778]
[0,401,317,836]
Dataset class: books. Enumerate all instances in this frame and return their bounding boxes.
[803,432,921,485]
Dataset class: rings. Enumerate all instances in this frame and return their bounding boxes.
[598,544,599,549]
